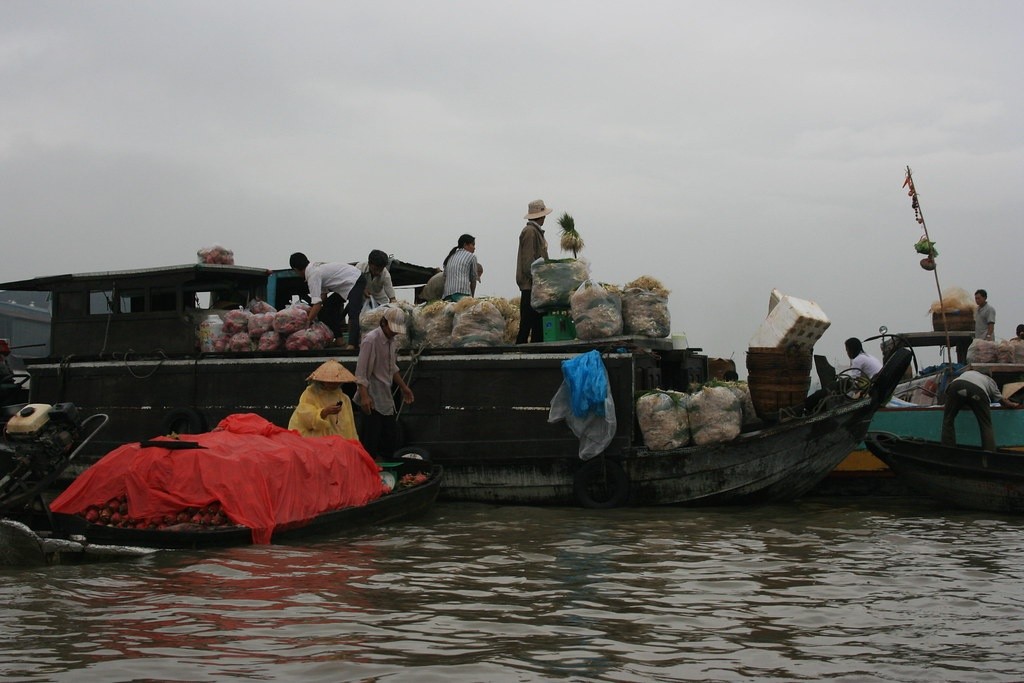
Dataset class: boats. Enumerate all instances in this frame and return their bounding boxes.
[0,258,919,511]
[862,361,1024,519]
[804,329,974,479]
[0,400,444,551]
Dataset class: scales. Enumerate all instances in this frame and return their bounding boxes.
[535,305,577,342]
[374,461,404,493]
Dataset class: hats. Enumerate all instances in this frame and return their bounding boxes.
[304,358,357,383]
[523,200,553,219]
[384,307,406,334]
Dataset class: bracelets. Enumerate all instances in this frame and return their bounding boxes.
[987,335,991,338]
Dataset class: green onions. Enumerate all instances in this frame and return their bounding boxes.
[359,296,521,346]
[633,379,763,451]
[557,212,584,252]
[530,258,671,339]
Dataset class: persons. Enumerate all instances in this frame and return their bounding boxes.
[421,234,483,304]
[290,252,367,350]
[973,289,997,342]
[1010,324,1024,341]
[355,250,397,309]
[516,199,553,344]
[942,367,1019,448]
[288,359,358,440]
[351,307,414,462]
[845,338,883,379]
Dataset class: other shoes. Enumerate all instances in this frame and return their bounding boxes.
[325,342,346,349]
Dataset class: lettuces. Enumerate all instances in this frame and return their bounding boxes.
[914,238,938,257]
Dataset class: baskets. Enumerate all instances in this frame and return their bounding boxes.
[745,343,813,421]
[933,309,973,331]
